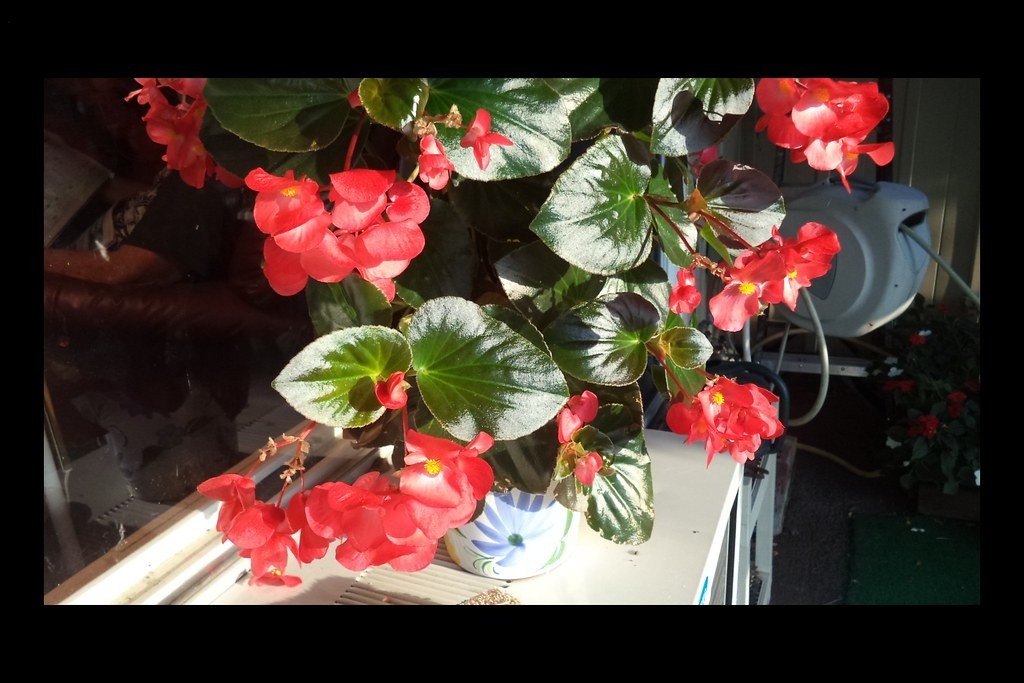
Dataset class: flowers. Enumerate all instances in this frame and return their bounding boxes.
[120,79,894,588]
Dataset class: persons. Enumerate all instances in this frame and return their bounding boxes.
[40,74,230,289]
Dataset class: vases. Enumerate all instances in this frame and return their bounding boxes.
[443,485,578,580]
